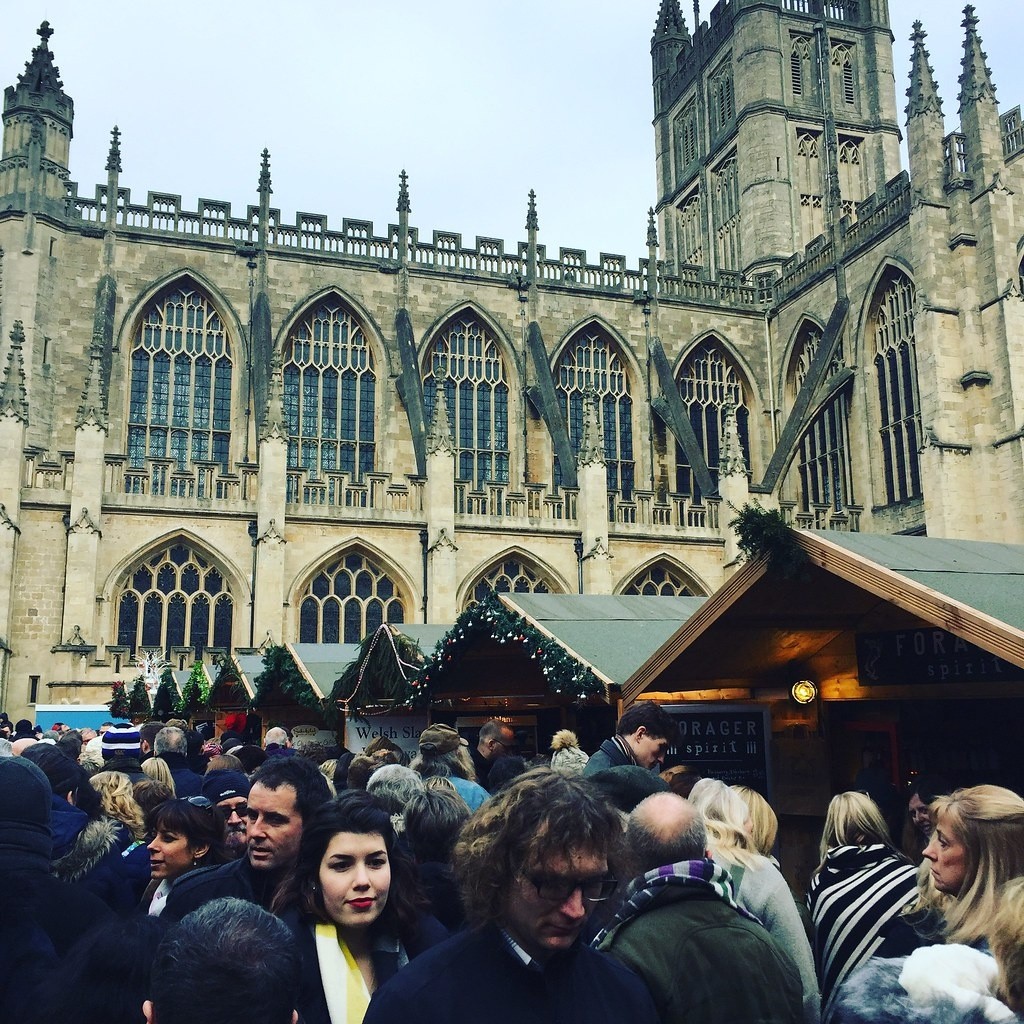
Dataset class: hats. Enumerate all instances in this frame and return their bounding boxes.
[22,743,84,793]
[0,756,52,826]
[42,730,58,742]
[16,720,31,732]
[102,722,139,760]
[420,724,471,757]
[202,769,250,804]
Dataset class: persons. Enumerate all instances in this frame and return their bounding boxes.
[0,719,1024,1024]
[582,701,678,774]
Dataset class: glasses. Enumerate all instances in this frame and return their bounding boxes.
[183,796,214,812]
[521,866,619,902]
[217,802,249,820]
[493,739,511,752]
